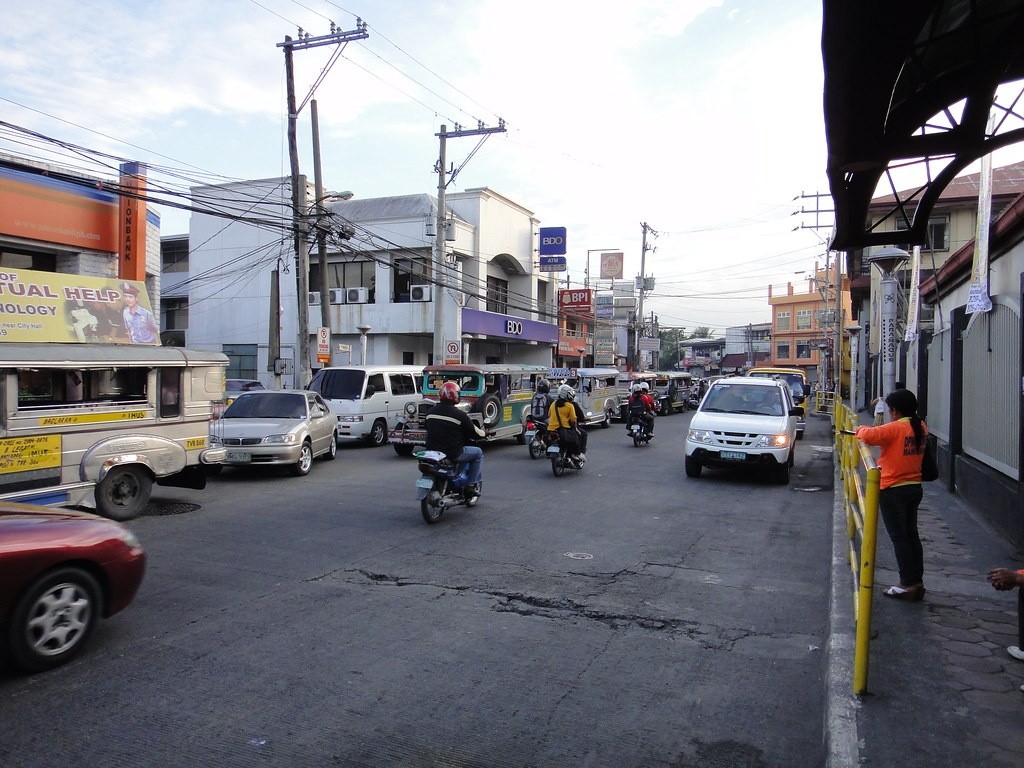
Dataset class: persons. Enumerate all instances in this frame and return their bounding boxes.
[531,379,588,461]
[756,390,782,415]
[854,389,927,601]
[786,377,803,396]
[626,382,656,437]
[809,379,836,397]
[987,568,1024,691]
[64,281,157,344]
[424,382,484,496]
[871,397,884,414]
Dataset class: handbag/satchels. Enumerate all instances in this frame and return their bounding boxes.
[558,427,578,446]
[920,432,938,481]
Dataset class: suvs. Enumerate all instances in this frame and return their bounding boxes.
[744,367,812,439]
[683,375,805,485]
[691,376,734,411]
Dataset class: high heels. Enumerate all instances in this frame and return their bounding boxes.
[882,582,925,601]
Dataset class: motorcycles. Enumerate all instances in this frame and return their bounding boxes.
[547,411,594,477]
[627,409,655,448]
[411,418,497,525]
[523,414,552,460]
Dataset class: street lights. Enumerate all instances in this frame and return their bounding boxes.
[868,244,913,432]
[293,175,358,386]
[846,325,863,413]
[461,333,472,385]
[577,347,587,369]
[818,343,829,412]
[355,323,374,366]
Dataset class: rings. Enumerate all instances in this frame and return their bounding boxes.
[994,573,995,577]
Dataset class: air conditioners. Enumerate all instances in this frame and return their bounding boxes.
[347,287,369,303]
[329,288,346,304]
[308,291,321,305]
[410,284,431,301]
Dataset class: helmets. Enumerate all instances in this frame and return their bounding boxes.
[632,384,642,393]
[537,379,550,393]
[558,384,570,397]
[560,386,575,400]
[439,381,460,403]
[640,382,649,392]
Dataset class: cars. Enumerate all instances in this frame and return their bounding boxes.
[225,378,267,405]
[212,389,340,475]
[0,500,145,671]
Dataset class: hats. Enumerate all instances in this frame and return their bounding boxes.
[119,282,140,297]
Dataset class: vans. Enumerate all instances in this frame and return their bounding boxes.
[302,365,430,445]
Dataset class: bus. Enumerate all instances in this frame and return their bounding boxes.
[653,372,692,416]
[386,364,550,460]
[550,367,621,429]
[618,372,659,424]
[0,341,230,524]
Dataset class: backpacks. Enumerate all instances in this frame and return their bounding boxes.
[632,395,643,416]
[532,393,547,418]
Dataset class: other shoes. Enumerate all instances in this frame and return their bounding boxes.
[648,432,654,437]
[466,486,481,497]
[579,453,586,462]
[1007,646,1024,661]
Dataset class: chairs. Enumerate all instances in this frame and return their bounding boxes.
[462,381,478,391]
